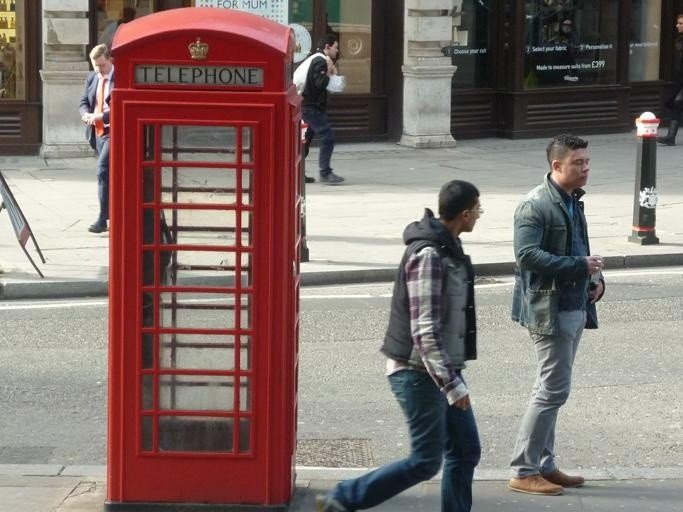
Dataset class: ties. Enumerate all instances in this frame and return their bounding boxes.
[95,79,107,137]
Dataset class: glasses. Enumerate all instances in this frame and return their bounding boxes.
[469,208,485,216]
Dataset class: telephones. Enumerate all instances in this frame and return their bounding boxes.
[143,194,172,285]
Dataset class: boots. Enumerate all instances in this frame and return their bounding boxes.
[657,120,679,146]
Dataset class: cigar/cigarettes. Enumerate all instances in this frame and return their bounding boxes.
[595,259,602,264]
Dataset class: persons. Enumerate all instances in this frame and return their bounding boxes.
[311,181,482,511]
[509,131,606,497]
[79,42,116,236]
[654,14,682,146]
[297,33,345,185]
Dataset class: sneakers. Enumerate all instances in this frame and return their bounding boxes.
[316,493,328,511]
[508,474,563,495]
[320,173,344,183]
[89,222,107,233]
[541,469,584,487]
[305,177,314,183]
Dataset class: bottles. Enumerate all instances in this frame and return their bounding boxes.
[590,259,601,290]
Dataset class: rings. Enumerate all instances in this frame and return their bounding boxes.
[594,266,599,270]
